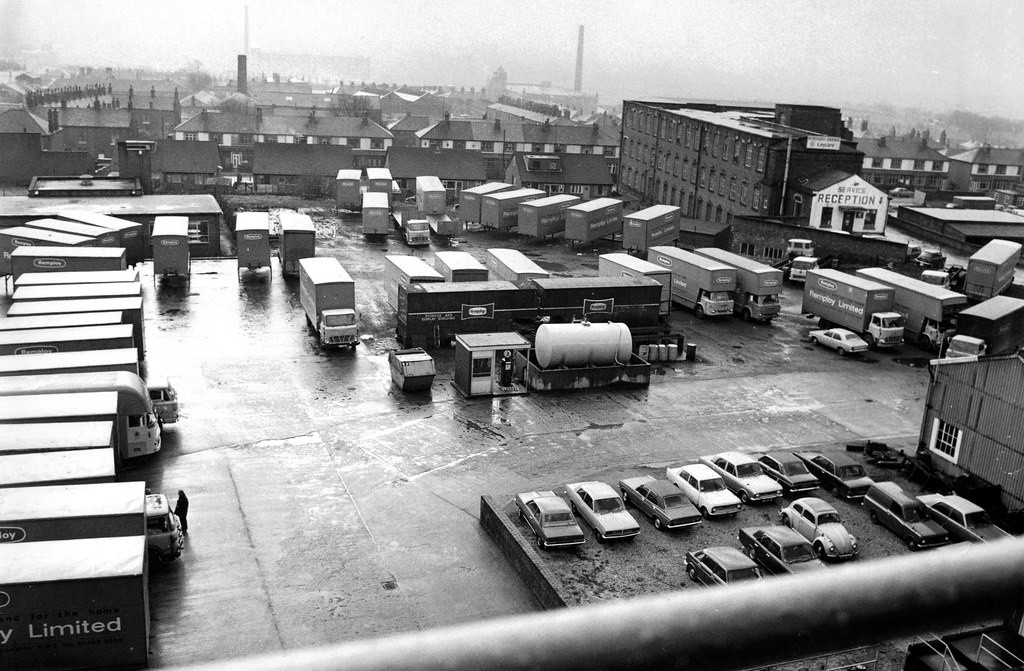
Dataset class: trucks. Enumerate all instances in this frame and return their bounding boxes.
[0,164,787,671]
[789,253,839,285]
[943,295,1024,361]
[946,196,997,210]
[802,267,969,349]
[964,239,1023,303]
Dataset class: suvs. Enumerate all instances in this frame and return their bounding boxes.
[865,481,950,551]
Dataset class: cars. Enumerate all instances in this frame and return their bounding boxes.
[570,480,641,542]
[753,449,821,493]
[809,328,869,356]
[666,463,743,519]
[686,545,762,590]
[517,490,586,549]
[739,524,828,576]
[918,492,1013,545]
[778,497,860,562]
[619,473,704,531]
[701,450,784,505]
[907,243,948,267]
[796,449,876,500]
[890,186,914,196]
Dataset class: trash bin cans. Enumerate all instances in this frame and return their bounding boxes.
[686,343,697,362]
